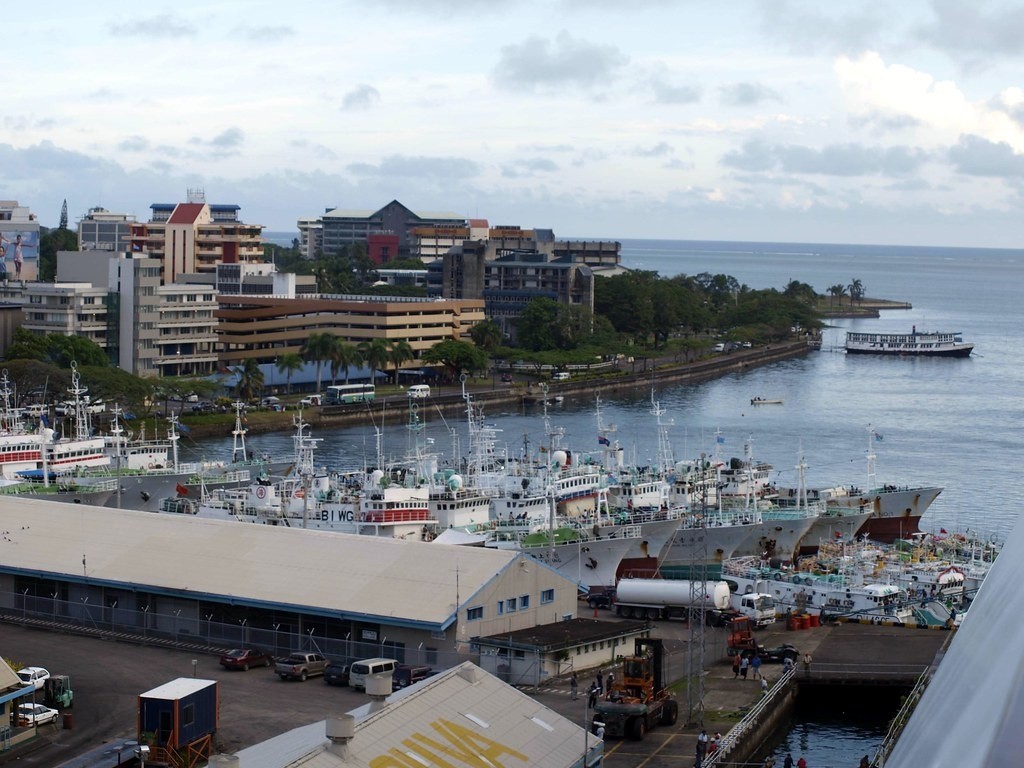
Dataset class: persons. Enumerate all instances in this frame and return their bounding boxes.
[693,729,721,768]
[761,676,768,690]
[804,653,813,673]
[732,652,762,680]
[782,655,794,675]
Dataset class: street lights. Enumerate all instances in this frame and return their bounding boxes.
[582,691,606,768]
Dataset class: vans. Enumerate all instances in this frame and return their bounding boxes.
[406,384,430,399]
[84,402,105,414]
[21,404,50,418]
[348,658,400,688]
[301,395,322,406]
[55,403,76,416]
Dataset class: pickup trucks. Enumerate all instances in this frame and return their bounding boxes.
[273,652,332,682]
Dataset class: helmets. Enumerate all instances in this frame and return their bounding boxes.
[609,672,614,675]
[710,737,715,741]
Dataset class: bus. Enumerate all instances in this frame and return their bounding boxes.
[326,384,376,406]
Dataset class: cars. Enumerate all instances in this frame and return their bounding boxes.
[323,658,365,687]
[715,341,752,352]
[219,647,273,671]
[192,401,210,411]
[8,667,51,694]
[586,588,616,609]
[9,702,60,729]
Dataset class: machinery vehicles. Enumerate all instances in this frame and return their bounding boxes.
[590,637,680,742]
[720,608,800,664]
[41,674,74,714]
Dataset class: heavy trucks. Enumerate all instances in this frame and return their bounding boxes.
[613,576,777,632]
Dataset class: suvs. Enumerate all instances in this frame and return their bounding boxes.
[392,665,433,691]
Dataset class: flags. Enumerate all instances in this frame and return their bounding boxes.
[875,432,884,441]
[177,422,192,433]
[598,436,611,447]
[716,435,725,443]
[941,527,948,533]
[124,413,137,419]
[834,528,843,536]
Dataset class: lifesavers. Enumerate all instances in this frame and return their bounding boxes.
[793,576,800,583]
[872,572,879,579]
[620,519,626,525]
[910,590,916,597]
[793,593,798,598]
[805,577,813,586]
[774,573,781,581]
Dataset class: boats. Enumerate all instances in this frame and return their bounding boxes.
[0,357,1007,629]
[843,324,975,358]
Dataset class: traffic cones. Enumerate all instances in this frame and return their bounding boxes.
[593,608,599,619]
